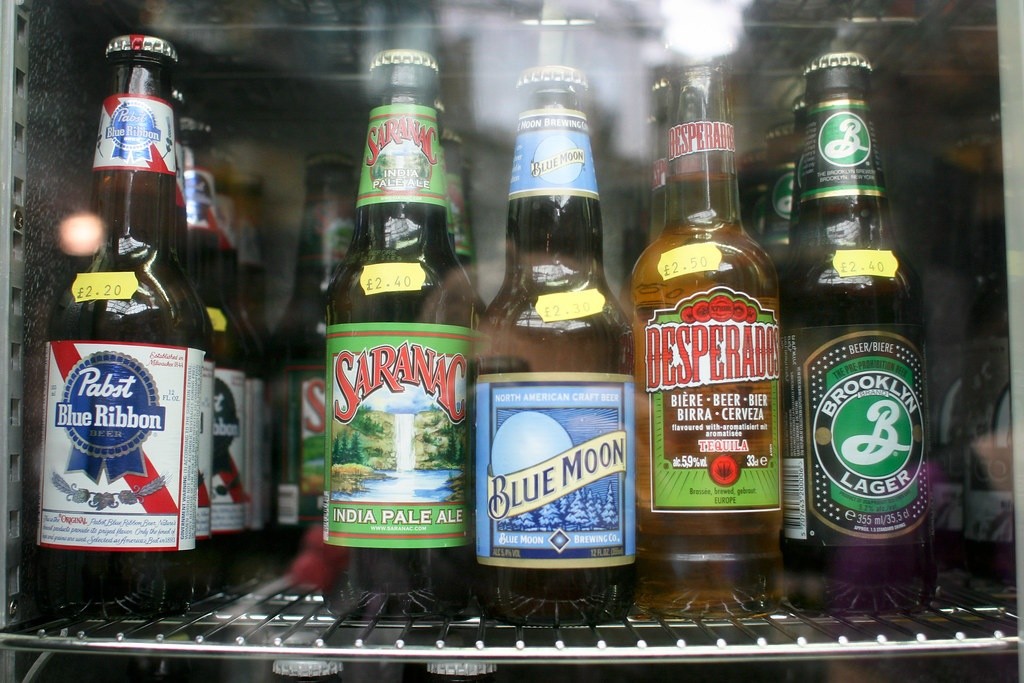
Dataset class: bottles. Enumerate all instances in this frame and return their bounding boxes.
[17,35,935,683]
